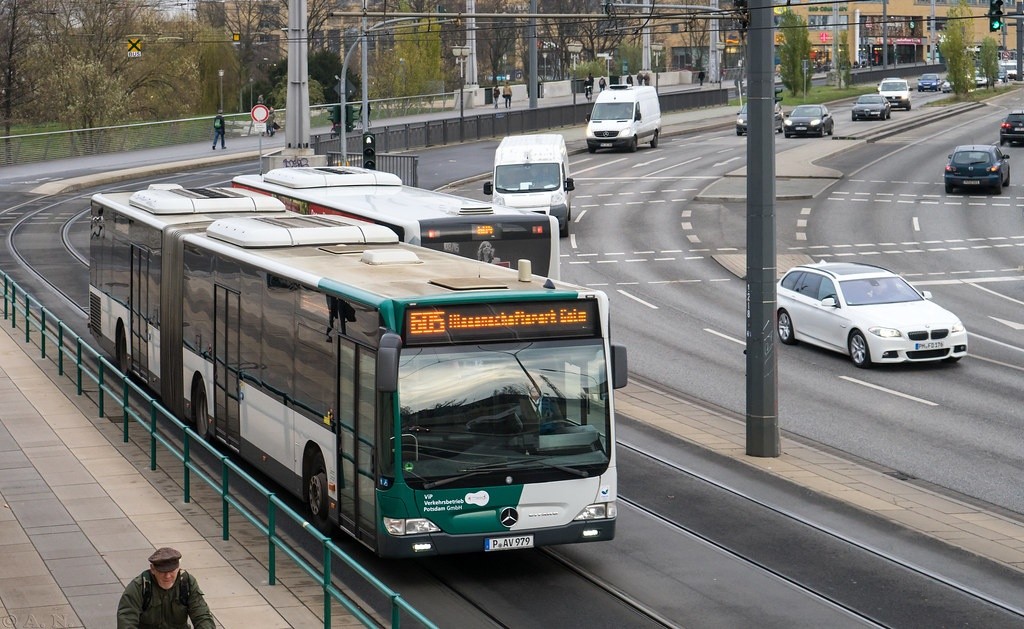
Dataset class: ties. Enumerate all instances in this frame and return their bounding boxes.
[530,398,541,420]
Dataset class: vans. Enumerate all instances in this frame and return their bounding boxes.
[484,133,575,237]
[584,84,661,153]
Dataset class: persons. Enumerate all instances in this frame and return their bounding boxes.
[116,548,216,629]
[514,369,565,434]
[503,82,512,108]
[627,73,633,86]
[644,72,650,86]
[584,73,594,97]
[266,108,276,137]
[698,70,705,86]
[637,73,643,86]
[359,102,371,127]
[212,110,226,150]
[477,241,500,264]
[494,85,500,109]
[599,75,606,92]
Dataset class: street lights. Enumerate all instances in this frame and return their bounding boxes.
[249,73,255,108]
[716,42,726,89]
[567,41,583,105]
[218,69,225,110]
[542,52,548,81]
[450,44,470,117]
[650,42,664,95]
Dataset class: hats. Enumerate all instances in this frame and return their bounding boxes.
[148,548,182,573]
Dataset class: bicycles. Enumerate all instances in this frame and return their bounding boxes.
[357,116,371,129]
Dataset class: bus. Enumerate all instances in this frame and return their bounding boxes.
[231,166,560,282]
[89,183,628,558]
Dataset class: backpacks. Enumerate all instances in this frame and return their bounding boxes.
[214,118,222,129]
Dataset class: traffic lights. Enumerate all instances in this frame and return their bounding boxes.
[363,132,376,170]
[327,105,340,133]
[990,0,1003,32]
[346,104,359,132]
[775,86,783,104]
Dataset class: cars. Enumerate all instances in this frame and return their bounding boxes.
[941,63,1017,93]
[784,104,834,138]
[944,145,1010,194]
[918,74,941,92]
[1000,110,1024,147]
[876,78,913,111]
[852,94,891,121]
[736,102,784,136]
[776,259,969,369]
